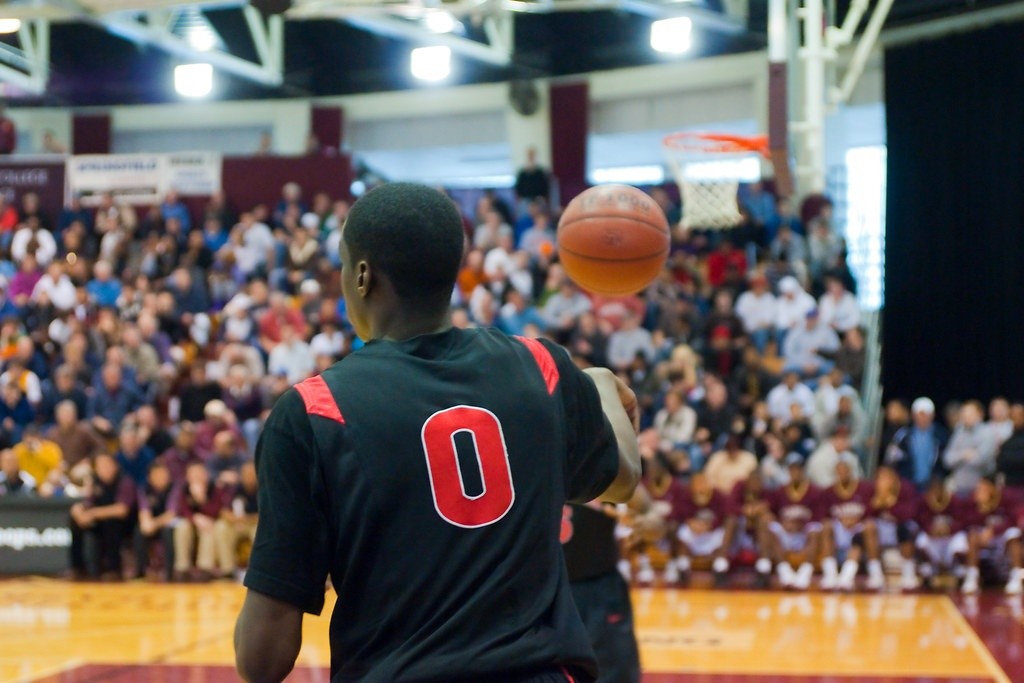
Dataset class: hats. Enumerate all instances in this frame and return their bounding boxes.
[778,275,798,296]
[911,398,935,415]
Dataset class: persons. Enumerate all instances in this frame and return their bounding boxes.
[234,182,641,683]
[0,148,1024,683]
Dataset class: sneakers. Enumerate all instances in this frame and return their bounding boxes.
[616,559,1023,596]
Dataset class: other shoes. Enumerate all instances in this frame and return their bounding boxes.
[84,564,234,582]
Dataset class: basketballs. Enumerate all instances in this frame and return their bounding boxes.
[556,180,672,298]
